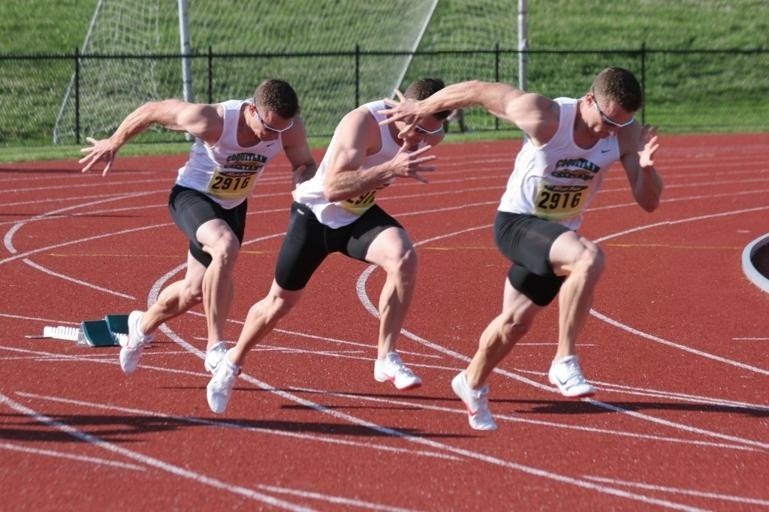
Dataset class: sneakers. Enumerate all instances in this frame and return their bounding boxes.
[548,355,596,398]
[452,371,499,431]
[119,308,152,378]
[372,352,423,391]
[206,357,240,414]
[203,341,243,377]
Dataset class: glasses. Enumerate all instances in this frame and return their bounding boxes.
[254,110,295,135]
[414,119,444,137]
[594,95,636,128]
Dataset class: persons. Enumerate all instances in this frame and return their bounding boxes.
[205,78,452,414]
[78,78,318,375]
[376,68,664,429]
